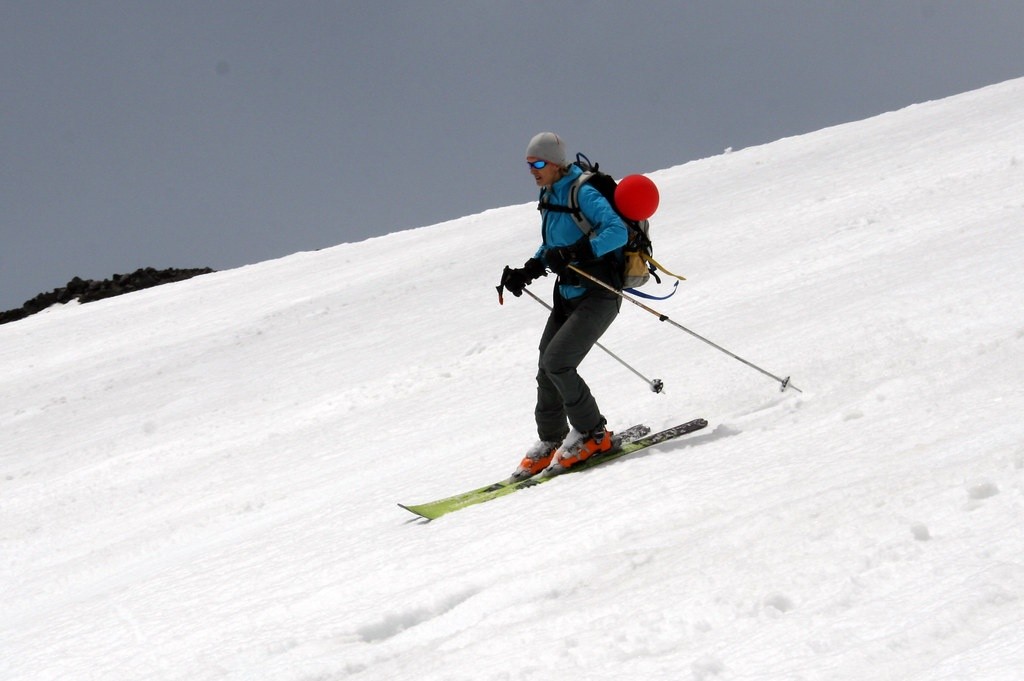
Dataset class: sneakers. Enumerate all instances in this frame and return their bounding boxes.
[516,438,562,475]
[554,418,615,468]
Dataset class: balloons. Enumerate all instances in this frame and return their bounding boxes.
[615,174,659,221]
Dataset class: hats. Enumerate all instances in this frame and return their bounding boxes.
[525,133,566,167]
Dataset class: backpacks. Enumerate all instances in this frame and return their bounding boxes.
[537,152,686,300]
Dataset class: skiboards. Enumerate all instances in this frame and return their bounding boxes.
[396,417,709,520]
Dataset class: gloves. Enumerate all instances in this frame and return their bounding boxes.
[546,235,596,276]
[505,257,548,297]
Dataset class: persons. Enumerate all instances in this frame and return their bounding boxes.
[503,132,629,482]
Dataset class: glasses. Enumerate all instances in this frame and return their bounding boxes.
[527,160,548,169]
